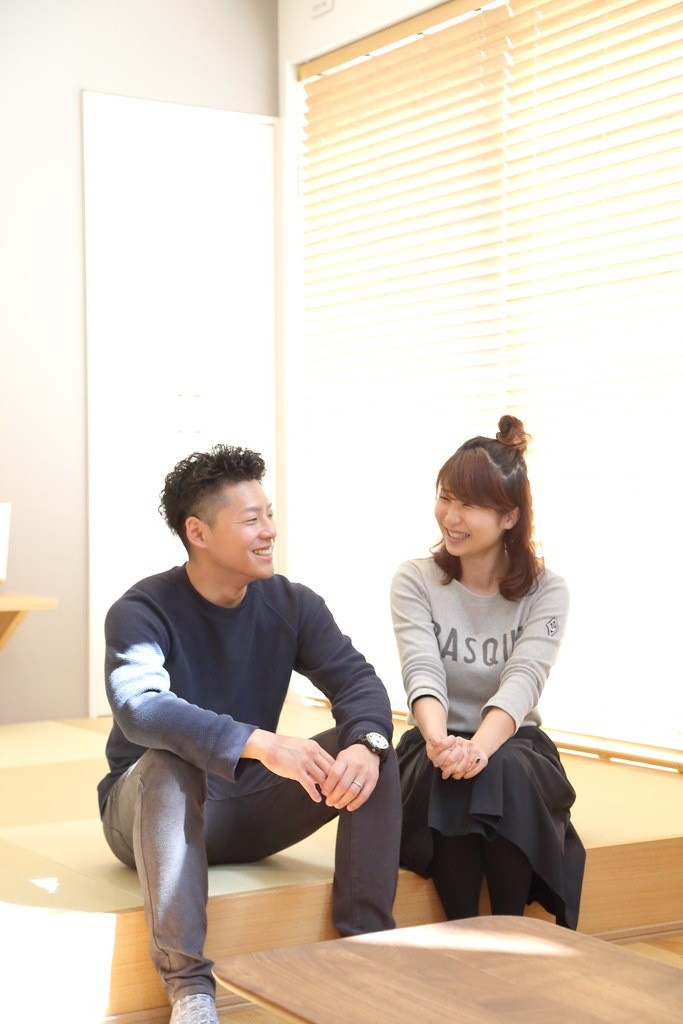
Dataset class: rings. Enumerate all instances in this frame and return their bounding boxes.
[352,781,362,788]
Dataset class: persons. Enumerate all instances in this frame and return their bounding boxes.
[96,444,402,1024]
[390,416,586,931]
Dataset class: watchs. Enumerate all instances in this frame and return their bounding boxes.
[352,732,390,772]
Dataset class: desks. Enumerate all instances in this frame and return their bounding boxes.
[211,914,683,1024]
